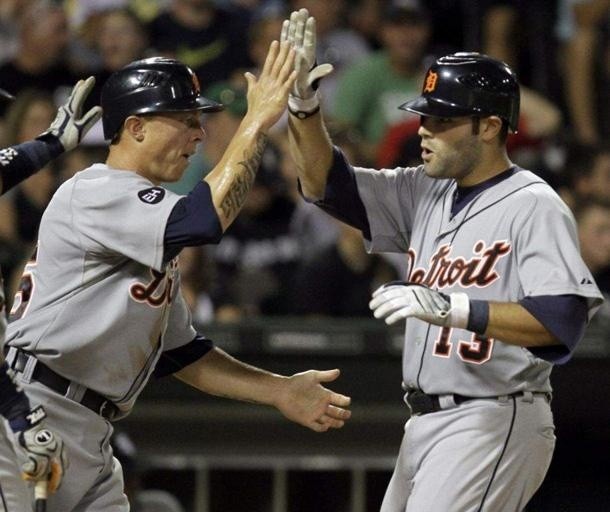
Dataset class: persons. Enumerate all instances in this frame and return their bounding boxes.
[0,36,356,511]
[275,6,607,511]
[0,72,108,495]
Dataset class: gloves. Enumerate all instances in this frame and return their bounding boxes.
[365,276,471,330]
[5,409,72,499]
[273,5,334,122]
[44,77,103,154]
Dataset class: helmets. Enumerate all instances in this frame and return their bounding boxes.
[103,53,226,145]
[395,50,523,137]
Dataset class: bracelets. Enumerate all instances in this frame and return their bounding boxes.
[467,299,491,335]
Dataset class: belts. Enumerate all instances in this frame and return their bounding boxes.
[402,380,530,415]
[0,339,123,425]
